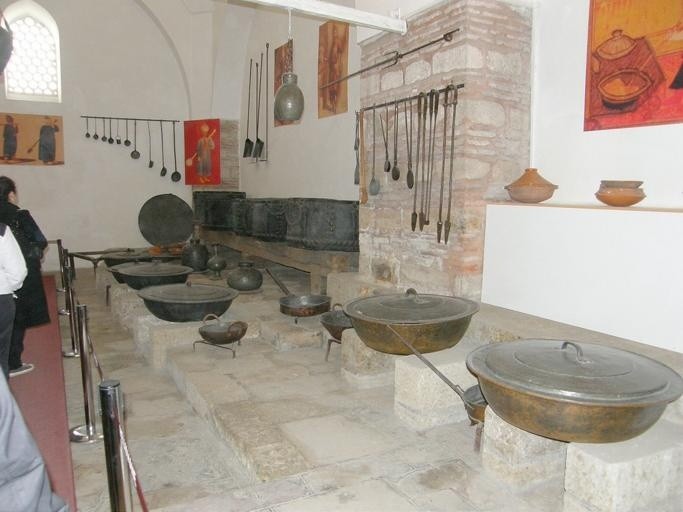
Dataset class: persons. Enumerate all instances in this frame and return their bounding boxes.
[0,213,32,381]
[36,116,59,165]
[193,123,215,183]
[0,176,51,379]
[0,115,19,161]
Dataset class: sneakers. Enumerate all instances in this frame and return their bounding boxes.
[9,363,35,378]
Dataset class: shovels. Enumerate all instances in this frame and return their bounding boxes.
[242,59,263,158]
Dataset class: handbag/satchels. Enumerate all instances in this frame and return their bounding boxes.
[10,226,44,260]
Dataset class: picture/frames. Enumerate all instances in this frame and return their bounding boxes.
[583,0,683,132]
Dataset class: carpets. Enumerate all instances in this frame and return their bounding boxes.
[0,275,76,512]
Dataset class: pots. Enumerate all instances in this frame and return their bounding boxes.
[345,289,481,357]
[104,248,353,351]
[465,338,683,443]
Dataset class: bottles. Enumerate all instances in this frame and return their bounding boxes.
[180,237,226,278]
[506,167,558,204]
